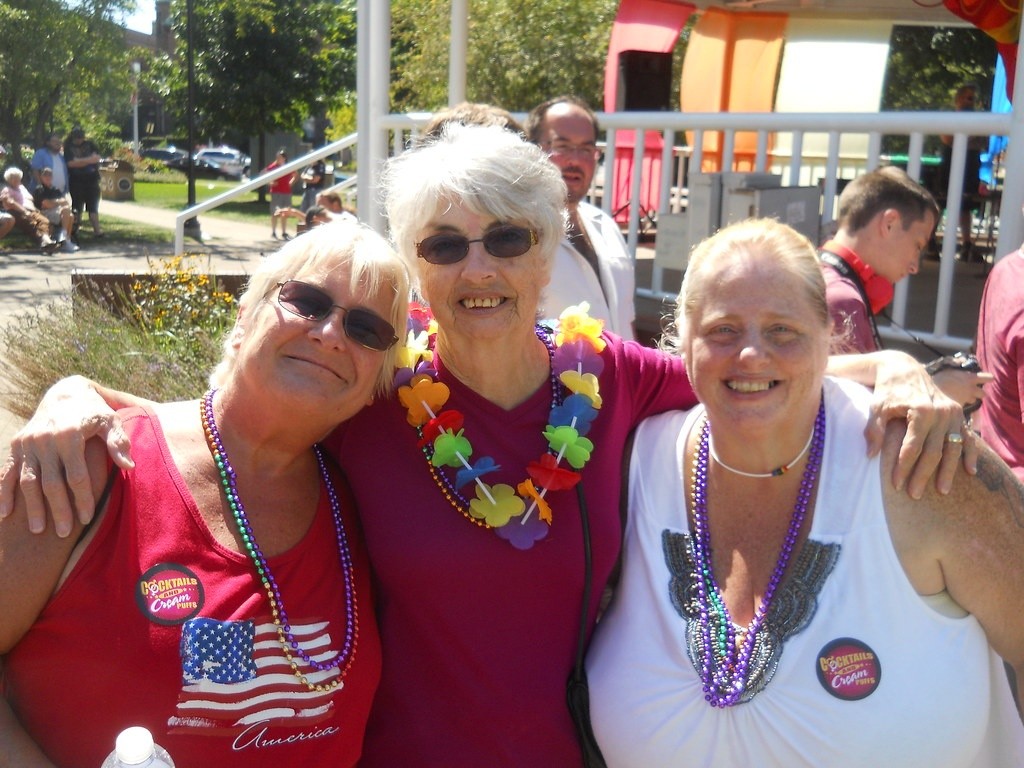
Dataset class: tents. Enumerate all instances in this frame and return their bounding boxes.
[605,0,1024,237]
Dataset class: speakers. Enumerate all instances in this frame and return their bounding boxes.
[614,50,673,112]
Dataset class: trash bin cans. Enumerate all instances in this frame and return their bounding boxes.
[99,158,116,199]
[107,159,135,202]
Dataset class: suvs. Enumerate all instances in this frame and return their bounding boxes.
[142,144,252,180]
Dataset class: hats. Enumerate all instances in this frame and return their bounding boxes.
[305,205,325,228]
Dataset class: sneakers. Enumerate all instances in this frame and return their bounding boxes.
[56,234,66,242]
[282,233,292,241]
[63,244,79,251]
[271,232,280,241]
[40,239,56,248]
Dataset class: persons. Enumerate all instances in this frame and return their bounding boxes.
[0,231,415,768]
[412,94,635,348]
[924,84,982,259]
[582,216,1024,767]
[811,164,939,352]
[0,120,978,768]
[976,240,1024,478]
[258,148,350,241]
[0,128,102,251]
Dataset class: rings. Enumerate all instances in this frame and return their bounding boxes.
[942,433,964,443]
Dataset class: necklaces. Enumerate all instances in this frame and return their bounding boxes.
[200,387,359,691]
[691,389,826,709]
[389,299,607,552]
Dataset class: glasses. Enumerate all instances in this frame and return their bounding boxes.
[416,226,538,265]
[263,279,400,352]
[549,143,598,157]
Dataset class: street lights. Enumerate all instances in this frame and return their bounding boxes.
[129,59,146,158]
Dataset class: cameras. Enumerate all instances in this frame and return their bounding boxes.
[922,351,983,414]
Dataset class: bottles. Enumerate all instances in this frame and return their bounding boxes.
[101,726,176,768]
